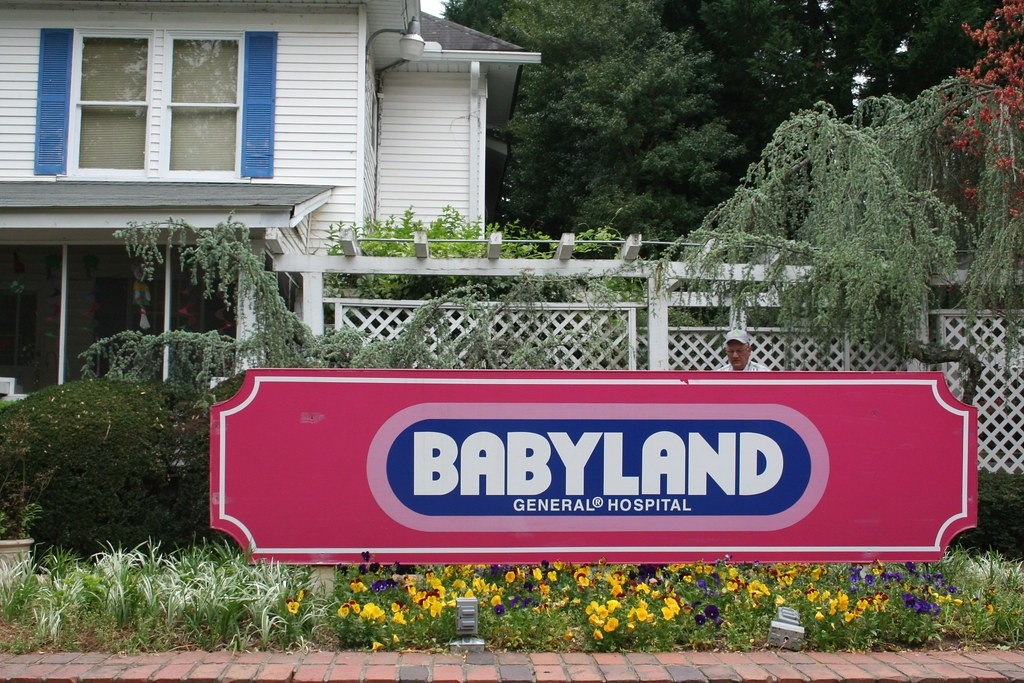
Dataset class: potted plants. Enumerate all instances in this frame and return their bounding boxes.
[0,414,60,572]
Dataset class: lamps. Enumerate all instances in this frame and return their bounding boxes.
[767,607,805,650]
[364,15,425,61]
[450,597,485,654]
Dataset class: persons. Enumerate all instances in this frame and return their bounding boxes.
[715,330,769,373]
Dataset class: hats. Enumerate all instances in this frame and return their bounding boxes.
[725,330,749,344]
